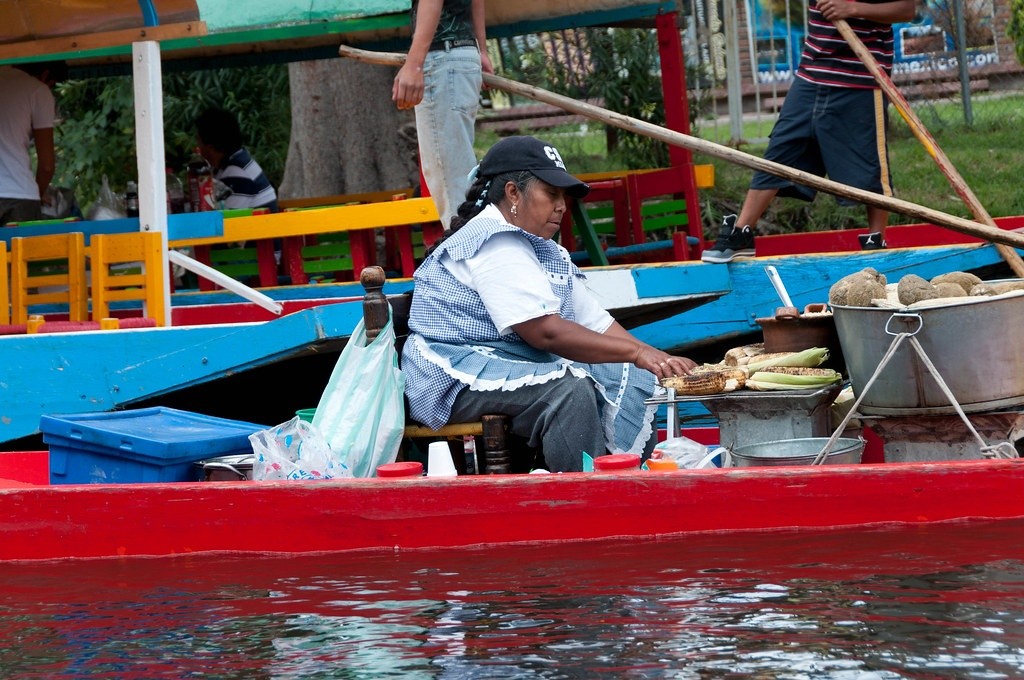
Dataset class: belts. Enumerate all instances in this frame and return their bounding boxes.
[429,38,477,51]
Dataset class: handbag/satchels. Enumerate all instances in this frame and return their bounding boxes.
[311,299,406,479]
[246,417,358,480]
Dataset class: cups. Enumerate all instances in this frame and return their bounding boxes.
[427,441,458,476]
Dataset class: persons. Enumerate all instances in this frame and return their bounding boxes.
[0,59,70,228]
[391,0,496,230]
[401,135,700,473]
[194,105,282,252]
[701,0,916,264]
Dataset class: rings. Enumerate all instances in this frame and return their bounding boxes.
[667,357,674,365]
[660,362,666,367]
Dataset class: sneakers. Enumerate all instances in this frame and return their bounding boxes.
[700,213,756,263]
[857,231,887,250]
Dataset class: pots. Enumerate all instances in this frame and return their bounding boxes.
[756,303,840,353]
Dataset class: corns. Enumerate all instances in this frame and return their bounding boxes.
[662,343,839,394]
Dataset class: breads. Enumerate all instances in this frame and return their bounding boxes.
[829,267,1024,307]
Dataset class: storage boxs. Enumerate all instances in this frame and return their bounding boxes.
[594,454,641,470]
[39,406,273,484]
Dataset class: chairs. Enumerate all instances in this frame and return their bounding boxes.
[360,266,545,476]
[0,231,167,335]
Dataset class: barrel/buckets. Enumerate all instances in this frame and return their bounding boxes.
[296,408,317,423]
[726,436,865,467]
[827,278,1024,416]
[193,454,255,481]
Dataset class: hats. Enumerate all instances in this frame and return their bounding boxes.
[476,136,591,201]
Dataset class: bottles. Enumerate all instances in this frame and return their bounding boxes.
[188,146,215,213]
[376,462,423,478]
[166,168,185,214]
[127,181,139,217]
[594,454,641,473]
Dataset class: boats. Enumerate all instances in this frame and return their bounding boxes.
[0,447,1024,560]
